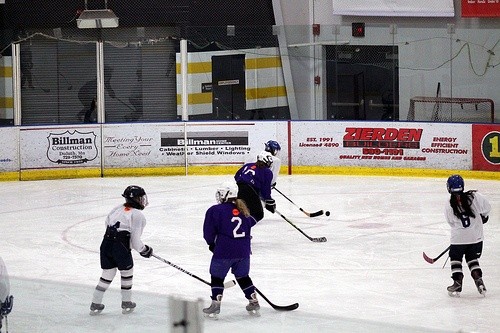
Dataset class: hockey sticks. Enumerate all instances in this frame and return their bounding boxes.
[274,209,327,242]
[423,245,451,264]
[252,284,299,311]
[151,253,236,288]
[273,186,324,217]
[5,315,9,333]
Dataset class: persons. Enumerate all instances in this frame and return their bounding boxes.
[203,186,260,319]
[445,175,492,298]
[90,185,153,314]
[20,49,35,88]
[244,140,281,187]
[167,41,180,76]
[0,256,14,333]
[77,67,115,121]
[234,152,276,229]
[124,71,143,119]
[84,98,97,122]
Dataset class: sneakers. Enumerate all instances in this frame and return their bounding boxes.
[121,301,137,315]
[475,278,487,295]
[202,294,223,320]
[245,292,261,318]
[89,302,105,316]
[446,280,462,298]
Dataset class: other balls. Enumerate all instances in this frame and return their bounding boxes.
[326,211,330,216]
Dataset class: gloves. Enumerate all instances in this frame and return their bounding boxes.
[264,198,276,214]
[271,181,277,189]
[140,245,153,258]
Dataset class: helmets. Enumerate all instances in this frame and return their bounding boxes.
[446,174,465,194]
[121,185,149,210]
[256,151,274,168]
[264,140,282,156]
[215,181,238,204]
